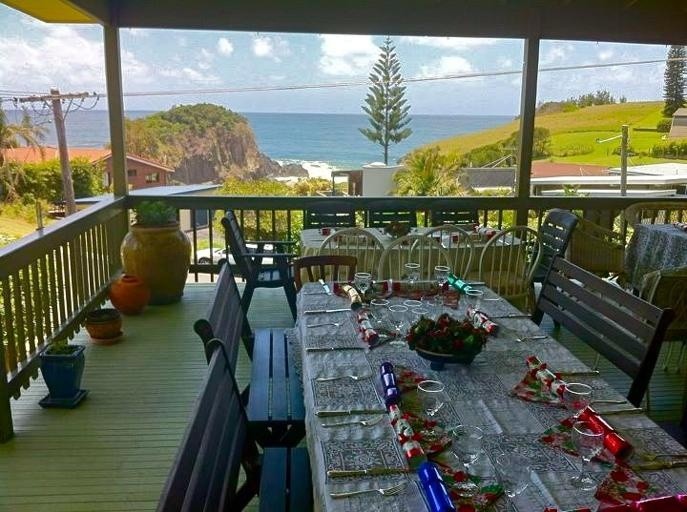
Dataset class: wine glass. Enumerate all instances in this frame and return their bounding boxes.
[569,420,605,491]
[416,379,446,442]
[494,452,533,511]
[451,424,485,498]
[353,272,371,309]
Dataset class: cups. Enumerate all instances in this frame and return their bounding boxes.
[465,289,484,311]
[370,297,445,348]
[404,262,420,282]
[434,266,450,286]
[563,381,592,419]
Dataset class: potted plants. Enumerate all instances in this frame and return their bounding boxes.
[119,199,191,305]
[84,308,121,339]
[36,337,90,410]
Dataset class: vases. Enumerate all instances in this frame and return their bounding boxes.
[109,274,147,315]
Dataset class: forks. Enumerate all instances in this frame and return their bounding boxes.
[329,482,408,499]
[322,416,384,428]
[316,374,371,382]
[305,320,345,328]
[631,451,687,461]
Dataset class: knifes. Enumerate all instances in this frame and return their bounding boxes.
[488,313,534,319]
[326,465,408,478]
[316,408,389,417]
[598,407,645,416]
[305,345,365,353]
[557,370,601,377]
[304,307,353,315]
[319,278,331,297]
[632,460,686,471]
